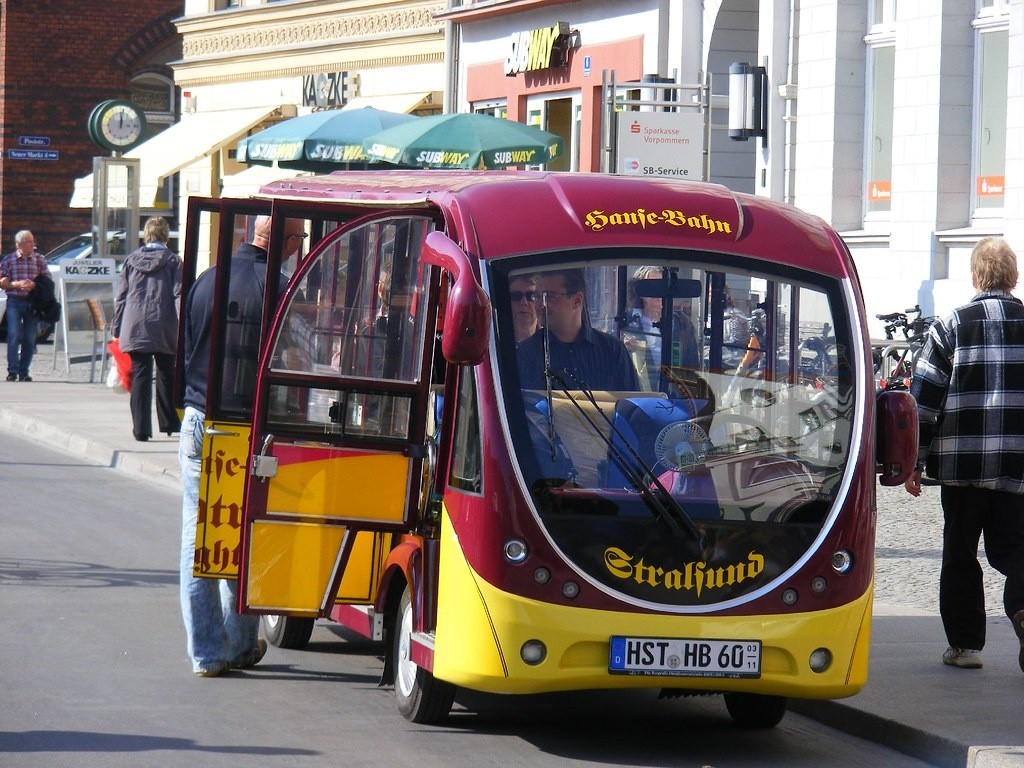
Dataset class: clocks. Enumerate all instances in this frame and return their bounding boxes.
[101,105,141,147]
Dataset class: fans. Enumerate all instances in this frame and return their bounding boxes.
[654,420,710,474]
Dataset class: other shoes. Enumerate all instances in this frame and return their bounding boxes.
[230,639,267,668]
[197,671,220,676]
[136,435,148,441]
[7,372,17,381]
[19,374,32,381]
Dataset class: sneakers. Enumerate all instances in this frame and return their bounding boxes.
[1013,610,1024,672]
[943,646,984,668]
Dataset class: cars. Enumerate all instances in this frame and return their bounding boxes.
[0,229,180,346]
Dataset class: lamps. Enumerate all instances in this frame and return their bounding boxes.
[727,61,770,150]
[639,73,677,113]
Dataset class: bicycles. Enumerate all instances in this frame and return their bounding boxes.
[722,305,941,394]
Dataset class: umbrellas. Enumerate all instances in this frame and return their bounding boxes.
[237,104,418,166]
[362,110,563,170]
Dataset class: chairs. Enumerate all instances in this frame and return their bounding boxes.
[86,298,111,385]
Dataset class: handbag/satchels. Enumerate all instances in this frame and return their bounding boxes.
[107,340,133,391]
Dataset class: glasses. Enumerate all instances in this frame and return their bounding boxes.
[510,291,535,302]
[285,233,308,240]
[537,293,573,302]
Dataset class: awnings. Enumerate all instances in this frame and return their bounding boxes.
[341,93,432,114]
[68,105,297,209]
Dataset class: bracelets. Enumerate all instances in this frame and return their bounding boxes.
[914,466,924,472]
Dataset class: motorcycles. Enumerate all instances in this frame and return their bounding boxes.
[177,168,922,727]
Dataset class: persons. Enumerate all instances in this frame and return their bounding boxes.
[177,213,302,678]
[905,237,1024,675]
[330,266,645,441]
[0,229,51,383]
[699,281,811,386]
[618,266,704,391]
[110,216,194,443]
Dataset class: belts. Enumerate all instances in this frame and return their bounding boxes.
[8,295,31,300]
